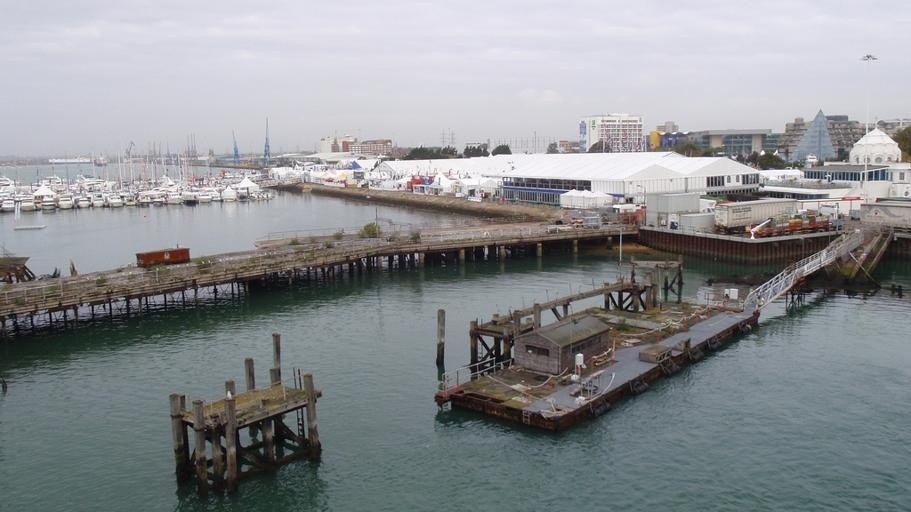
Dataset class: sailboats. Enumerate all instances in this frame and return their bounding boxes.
[0,133,267,213]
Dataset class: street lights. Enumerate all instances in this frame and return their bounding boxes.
[853,52,880,196]
